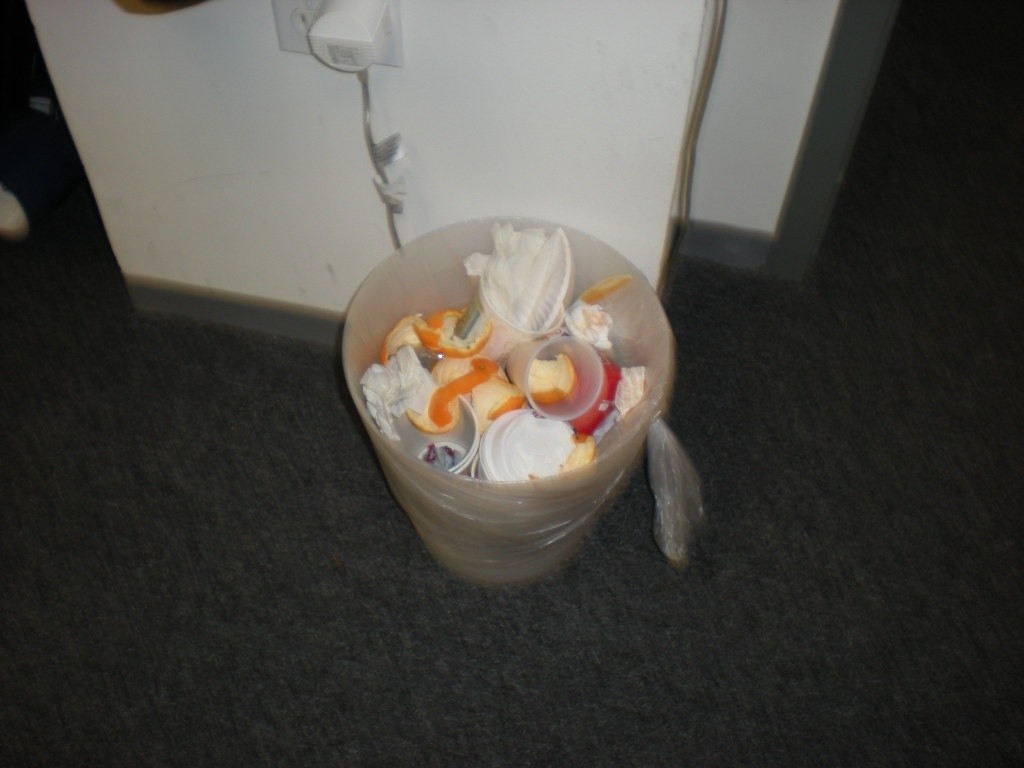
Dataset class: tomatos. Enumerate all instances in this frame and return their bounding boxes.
[570,348,624,435]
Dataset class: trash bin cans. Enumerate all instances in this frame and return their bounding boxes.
[340,217,679,589]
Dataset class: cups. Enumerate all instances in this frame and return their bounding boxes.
[386,385,586,487]
[507,335,604,421]
[456,264,567,365]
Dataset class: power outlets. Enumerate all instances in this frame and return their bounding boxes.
[272,0,403,67]
